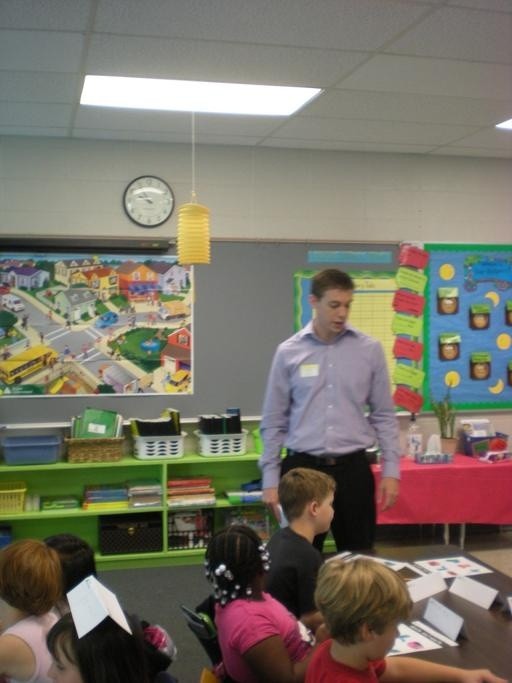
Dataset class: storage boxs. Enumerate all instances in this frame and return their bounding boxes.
[3,434,61,463]
[97,513,163,555]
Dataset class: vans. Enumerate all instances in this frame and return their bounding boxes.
[0,294,26,314]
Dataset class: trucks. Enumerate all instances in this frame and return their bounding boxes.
[159,298,190,321]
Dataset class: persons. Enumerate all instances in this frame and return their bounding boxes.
[260,267,402,554]
[304,558,510,683]
[49,609,152,680]
[0,537,63,681]
[259,466,337,616]
[202,523,328,679]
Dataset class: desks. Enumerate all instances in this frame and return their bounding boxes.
[369,454,511,549]
[242,547,511,683]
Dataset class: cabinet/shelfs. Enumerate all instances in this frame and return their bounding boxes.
[1,458,336,572]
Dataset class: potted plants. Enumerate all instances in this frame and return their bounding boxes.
[425,382,462,453]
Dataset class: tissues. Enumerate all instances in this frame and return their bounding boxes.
[414,434,453,465]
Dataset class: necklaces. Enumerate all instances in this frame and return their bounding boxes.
[43,532,97,598]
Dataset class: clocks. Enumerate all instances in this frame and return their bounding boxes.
[122,173,177,228]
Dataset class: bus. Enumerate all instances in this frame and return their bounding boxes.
[0,344,58,385]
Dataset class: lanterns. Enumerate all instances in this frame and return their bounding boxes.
[175,202,212,266]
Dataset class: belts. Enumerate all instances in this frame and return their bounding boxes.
[290,451,365,468]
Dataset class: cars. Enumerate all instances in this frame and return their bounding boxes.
[164,367,191,391]
[95,310,119,328]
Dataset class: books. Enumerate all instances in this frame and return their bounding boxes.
[82,470,216,511]
[71,401,242,437]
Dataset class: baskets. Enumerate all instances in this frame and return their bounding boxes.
[96,520,164,554]
[193,429,250,458]
[131,432,188,459]
[65,434,128,462]
[1,480,26,517]
[167,510,216,551]
[463,431,508,459]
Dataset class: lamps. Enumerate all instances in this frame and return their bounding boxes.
[177,111,210,268]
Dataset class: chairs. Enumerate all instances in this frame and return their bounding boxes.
[183,602,221,666]
